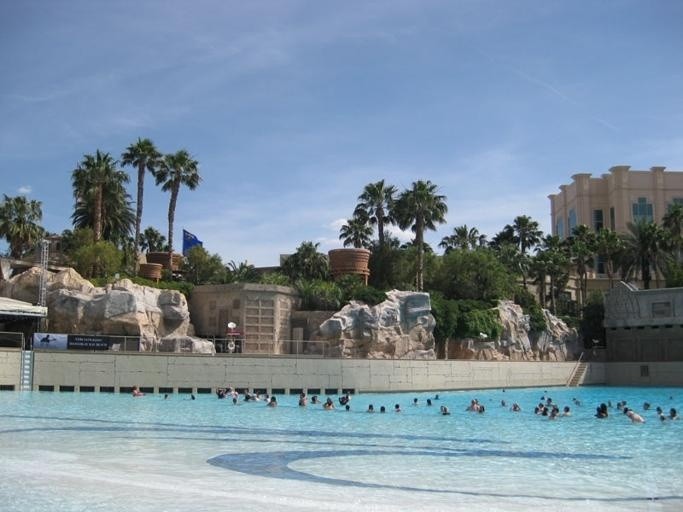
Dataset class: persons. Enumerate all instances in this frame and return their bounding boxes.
[535,390,572,420]
[130,386,144,396]
[644,402,678,422]
[512,403,520,410]
[470,398,484,412]
[300,392,350,411]
[414,395,448,414]
[594,400,645,423]
[215,387,276,407]
[368,404,400,414]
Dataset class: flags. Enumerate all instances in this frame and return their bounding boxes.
[183,228,204,258]
